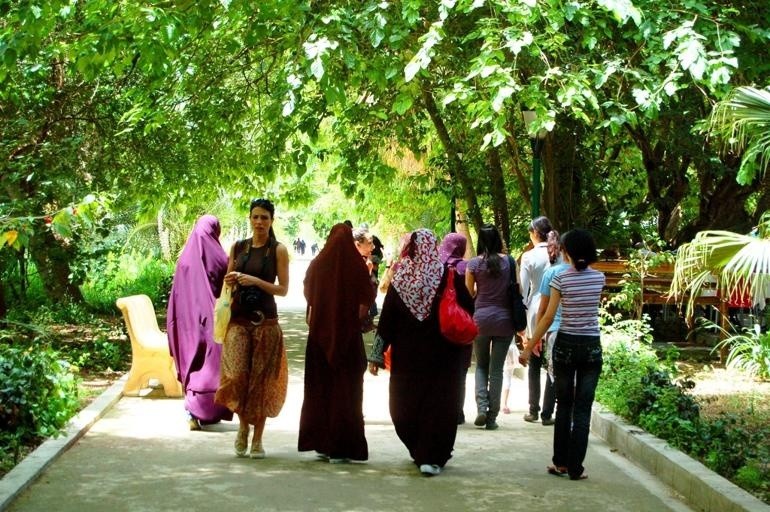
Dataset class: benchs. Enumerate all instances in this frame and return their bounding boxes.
[115,294,184,397]
[582,258,731,364]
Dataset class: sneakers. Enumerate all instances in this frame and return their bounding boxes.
[474,413,499,430]
[234,427,249,455]
[547,464,589,480]
[190,418,201,430]
[250,439,265,459]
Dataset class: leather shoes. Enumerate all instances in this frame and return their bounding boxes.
[524,413,538,422]
[419,464,442,475]
[542,418,557,426]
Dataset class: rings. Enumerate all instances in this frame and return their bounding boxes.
[239,280,241,284]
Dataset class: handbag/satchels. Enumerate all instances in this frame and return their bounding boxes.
[507,255,527,331]
[439,268,480,345]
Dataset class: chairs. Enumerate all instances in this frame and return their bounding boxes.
[717,272,770,334]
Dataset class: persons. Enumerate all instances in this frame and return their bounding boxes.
[344,220,384,321]
[519,229,611,480]
[216,199,292,457]
[367,230,475,476]
[293,236,319,257]
[436,233,473,424]
[514,216,561,425]
[489,333,519,414]
[597,231,704,327]
[297,222,378,462]
[528,231,578,427]
[166,215,233,430]
[464,224,524,428]
